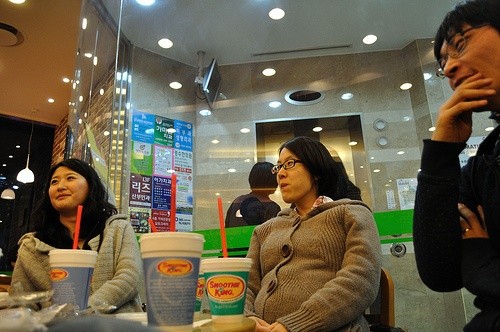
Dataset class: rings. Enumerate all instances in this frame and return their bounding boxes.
[463,228,472,233]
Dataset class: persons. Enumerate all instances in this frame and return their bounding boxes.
[413,0,500,332]
[219,161,281,257]
[245,137,382,332]
[8,158,147,314]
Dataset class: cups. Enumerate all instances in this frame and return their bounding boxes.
[137,231,207,332]
[47,248,99,310]
[192,259,206,321]
[199,257,255,325]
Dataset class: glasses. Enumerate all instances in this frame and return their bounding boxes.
[434,23,489,80]
[271,158,303,174]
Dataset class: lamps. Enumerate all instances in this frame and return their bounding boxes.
[1,187,16,200]
[16,124,34,184]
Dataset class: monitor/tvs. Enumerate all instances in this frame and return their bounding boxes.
[194,58,222,108]
